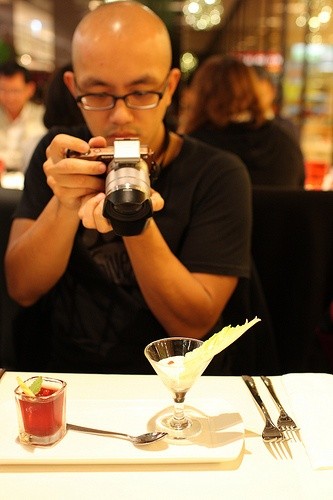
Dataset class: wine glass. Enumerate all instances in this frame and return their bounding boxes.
[145,338,214,440]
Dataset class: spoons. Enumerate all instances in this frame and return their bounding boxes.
[66,424,168,446]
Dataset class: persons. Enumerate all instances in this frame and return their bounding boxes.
[4,2,252,378]
[0,53,304,194]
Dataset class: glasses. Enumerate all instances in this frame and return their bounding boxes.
[73,74,170,111]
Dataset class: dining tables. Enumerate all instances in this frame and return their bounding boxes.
[0,372,333,500]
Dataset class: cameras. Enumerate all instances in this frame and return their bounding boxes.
[66,137,160,237]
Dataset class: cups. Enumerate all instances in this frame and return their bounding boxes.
[15,376,67,446]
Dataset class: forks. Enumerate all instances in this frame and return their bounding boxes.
[261,376,301,432]
[243,375,291,443]
